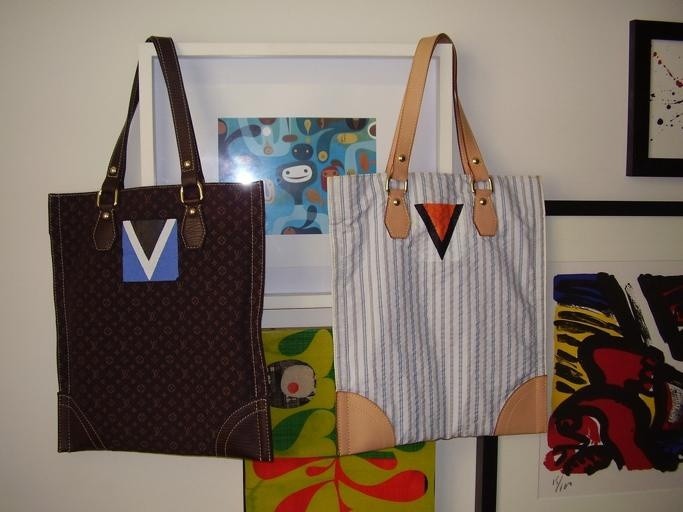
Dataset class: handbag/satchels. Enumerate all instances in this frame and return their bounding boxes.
[325,168,550,458]
[48,180,274,464]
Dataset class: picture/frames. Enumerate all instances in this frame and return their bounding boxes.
[135,40,453,314]
[625,18,683,178]
[473,199,683,512]
